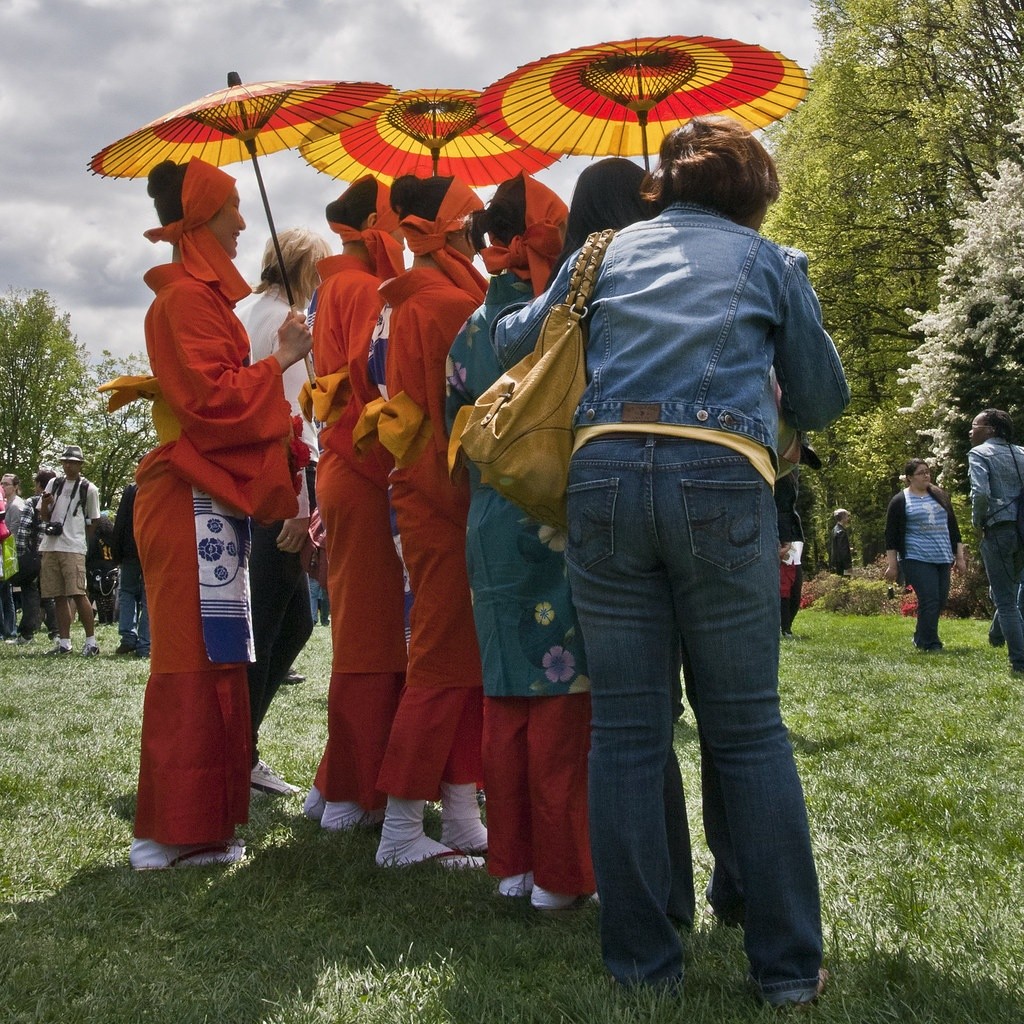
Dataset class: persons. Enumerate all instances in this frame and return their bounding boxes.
[966,409,1024,670]
[0,445,151,659]
[884,459,966,649]
[832,508,853,577]
[489,115,850,1009]
[774,465,805,638]
[233,157,664,905]
[96,157,313,869]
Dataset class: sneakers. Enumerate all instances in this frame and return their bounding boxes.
[250,759,302,795]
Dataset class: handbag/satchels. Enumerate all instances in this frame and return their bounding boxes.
[0,532,19,581]
[459,229,615,533]
[299,507,328,588]
[10,553,41,587]
[89,569,120,597]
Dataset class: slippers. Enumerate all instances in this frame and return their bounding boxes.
[537,894,599,912]
[412,848,483,869]
[130,838,246,870]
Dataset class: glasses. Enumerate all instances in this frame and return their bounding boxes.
[1,482,14,486]
[911,471,930,476]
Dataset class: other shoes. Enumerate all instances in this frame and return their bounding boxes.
[116,644,134,654]
[6,636,31,644]
[322,619,330,626]
[58,638,72,653]
[53,635,60,645]
[136,649,150,657]
[83,644,99,656]
[781,625,792,637]
[280,666,306,684]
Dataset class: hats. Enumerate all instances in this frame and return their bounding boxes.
[59,446,87,463]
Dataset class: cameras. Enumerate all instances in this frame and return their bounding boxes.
[45,522,63,536]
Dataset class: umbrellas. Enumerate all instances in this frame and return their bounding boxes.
[86,71,403,390]
[298,87,564,187]
[477,35,812,172]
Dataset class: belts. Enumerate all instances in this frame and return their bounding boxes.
[589,432,681,441]
